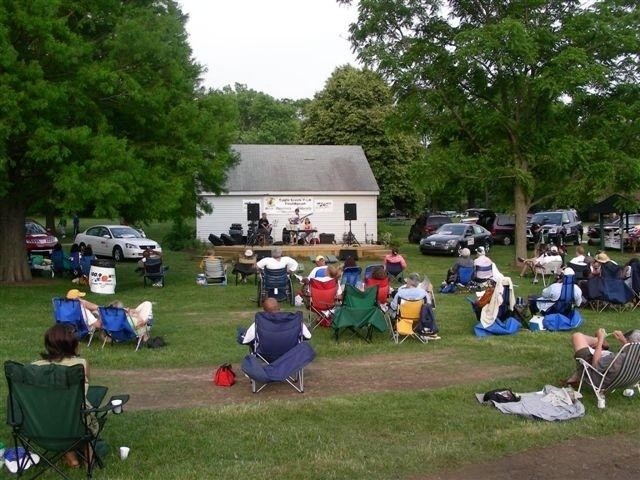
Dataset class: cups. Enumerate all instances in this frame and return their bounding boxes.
[119,445,131,461]
[110,399,123,415]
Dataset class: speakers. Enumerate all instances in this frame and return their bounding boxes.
[247,204,259,221]
[344,203,356,220]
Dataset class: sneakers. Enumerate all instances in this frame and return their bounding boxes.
[4,452,40,473]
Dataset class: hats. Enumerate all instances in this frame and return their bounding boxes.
[315,256,325,261]
[245,249,253,256]
[595,253,610,263]
[476,246,486,255]
[66,289,86,299]
[561,268,575,276]
[551,245,558,253]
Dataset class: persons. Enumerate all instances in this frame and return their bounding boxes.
[236,296,313,350]
[255,248,431,319]
[200,249,256,283]
[258,209,313,246]
[445,222,640,313]
[54,242,97,277]
[68,289,152,341]
[141,249,163,287]
[564,327,640,389]
[30,322,100,470]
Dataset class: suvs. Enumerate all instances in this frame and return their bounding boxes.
[408,214,453,244]
[464,207,538,246]
[529,208,585,249]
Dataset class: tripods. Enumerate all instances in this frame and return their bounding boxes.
[246,223,261,246]
[341,220,361,247]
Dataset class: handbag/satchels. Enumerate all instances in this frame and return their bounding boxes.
[417,295,438,336]
[215,363,236,387]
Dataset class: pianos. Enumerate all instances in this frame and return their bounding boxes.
[298,229,317,232]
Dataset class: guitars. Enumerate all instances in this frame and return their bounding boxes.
[290,213,312,224]
[260,224,271,234]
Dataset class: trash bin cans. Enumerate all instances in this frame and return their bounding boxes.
[89,260,116,294]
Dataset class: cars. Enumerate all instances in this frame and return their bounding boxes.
[456,213,469,218]
[587,213,640,239]
[74,224,163,262]
[419,223,494,257]
[24,219,60,259]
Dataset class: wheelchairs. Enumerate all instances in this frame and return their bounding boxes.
[257,265,296,308]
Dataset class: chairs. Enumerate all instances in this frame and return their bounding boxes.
[3,358,109,480]
[576,341,640,405]
